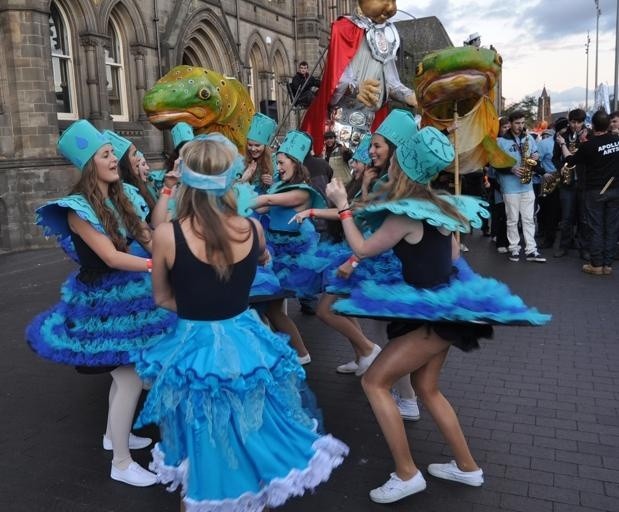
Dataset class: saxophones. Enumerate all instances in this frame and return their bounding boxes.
[519,129,537,185]
[563,128,578,186]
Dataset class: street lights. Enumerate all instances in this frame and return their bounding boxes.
[585,27,591,112]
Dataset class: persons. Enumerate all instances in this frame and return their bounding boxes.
[291,61,321,106]
[468,32,482,49]
[484,107,619,274]
[326,0,421,158]
[25,109,553,512]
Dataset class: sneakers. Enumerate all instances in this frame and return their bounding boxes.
[428,460,483,487]
[297,344,420,420]
[461,232,613,275]
[104,433,152,450]
[370,470,427,503]
[111,461,158,487]
